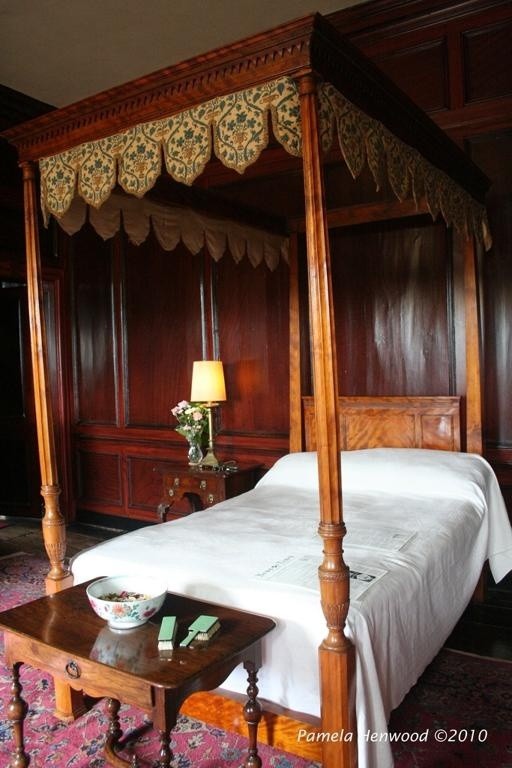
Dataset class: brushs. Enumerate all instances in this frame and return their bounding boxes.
[158,615,222,651]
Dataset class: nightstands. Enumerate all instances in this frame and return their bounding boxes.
[151,460,265,525]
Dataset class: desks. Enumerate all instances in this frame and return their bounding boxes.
[0,577,277,768]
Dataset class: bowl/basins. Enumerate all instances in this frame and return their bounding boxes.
[88,620,173,676]
[85,574,169,630]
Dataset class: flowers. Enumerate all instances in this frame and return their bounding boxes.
[172,403,209,459]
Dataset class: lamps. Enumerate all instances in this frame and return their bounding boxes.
[188,359,229,469]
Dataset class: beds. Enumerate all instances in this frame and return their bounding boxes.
[42,392,491,767]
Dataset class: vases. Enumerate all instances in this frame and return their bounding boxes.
[187,443,203,465]
